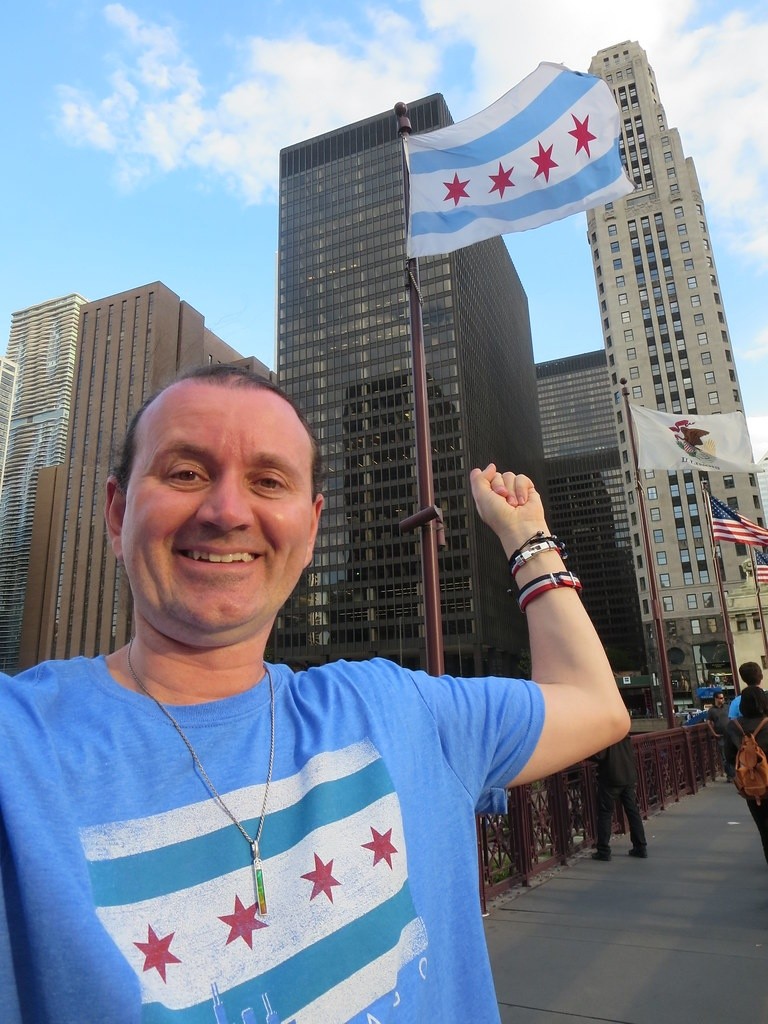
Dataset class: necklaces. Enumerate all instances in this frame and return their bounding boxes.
[128,639,274,916]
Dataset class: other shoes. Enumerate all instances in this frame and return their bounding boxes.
[592,847,611,861]
[629,847,647,858]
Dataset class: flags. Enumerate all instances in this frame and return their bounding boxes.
[754,548,768,583]
[629,403,764,473]
[400,62,637,260]
[709,495,768,546]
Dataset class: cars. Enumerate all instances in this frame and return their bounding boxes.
[676,708,702,721]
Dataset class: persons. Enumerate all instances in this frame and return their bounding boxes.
[724,662,768,865]
[0,364,632,1024]
[706,691,733,783]
[587,735,647,862]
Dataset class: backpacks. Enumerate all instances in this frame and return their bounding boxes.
[732,717,768,806]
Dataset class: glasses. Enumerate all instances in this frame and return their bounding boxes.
[717,698,723,699]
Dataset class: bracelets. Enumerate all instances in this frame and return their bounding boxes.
[516,571,582,617]
[509,531,568,579]
